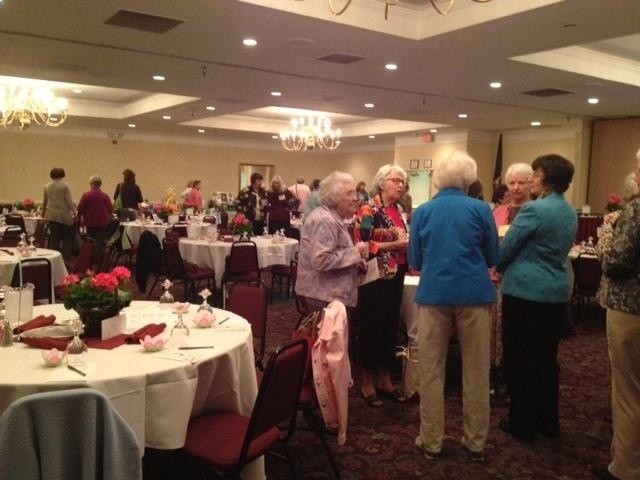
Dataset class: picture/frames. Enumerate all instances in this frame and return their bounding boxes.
[410,159,419,169]
[424,160,432,168]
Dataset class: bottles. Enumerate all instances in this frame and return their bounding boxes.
[0,316,15,348]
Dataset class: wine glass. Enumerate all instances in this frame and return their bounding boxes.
[159,278,174,306]
[197,288,215,328]
[170,311,189,348]
[572,235,597,257]
[65,318,88,365]
[1,201,303,258]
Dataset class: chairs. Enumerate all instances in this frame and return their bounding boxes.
[223,281,355,476]
[171,337,311,478]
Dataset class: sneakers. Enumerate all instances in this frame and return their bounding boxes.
[414,435,440,459]
[461,435,486,461]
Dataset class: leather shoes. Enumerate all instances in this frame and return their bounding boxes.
[362,388,407,406]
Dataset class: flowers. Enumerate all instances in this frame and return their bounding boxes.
[605,192,627,213]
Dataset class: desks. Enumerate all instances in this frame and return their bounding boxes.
[0,295,269,478]
[0,172,567,378]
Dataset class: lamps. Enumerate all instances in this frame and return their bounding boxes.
[279,109,342,152]
[0,75,69,131]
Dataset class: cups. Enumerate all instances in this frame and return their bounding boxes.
[360,242,370,259]
[344,215,358,225]
[0,282,35,326]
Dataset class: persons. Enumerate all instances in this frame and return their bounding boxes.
[40,167,77,262]
[487,162,532,284]
[113,169,142,223]
[587,149,640,480]
[182,178,203,214]
[293,164,412,406]
[233,173,311,236]
[409,150,500,460]
[490,155,579,445]
[77,175,113,267]
